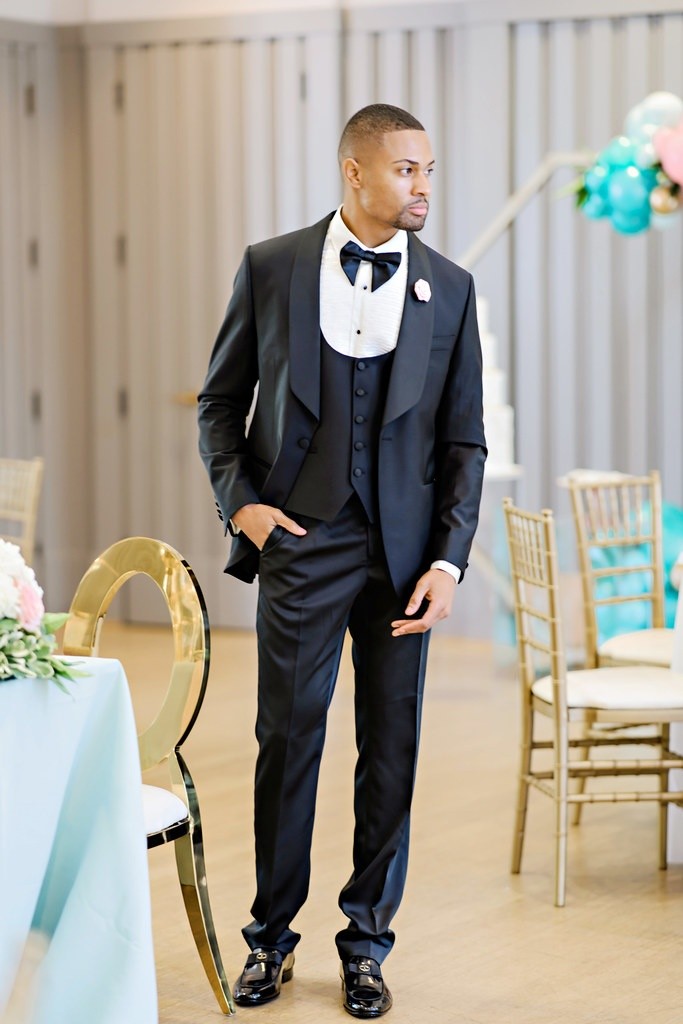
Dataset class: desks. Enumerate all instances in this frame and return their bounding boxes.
[0,653,161,1024]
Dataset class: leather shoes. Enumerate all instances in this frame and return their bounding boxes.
[343,955,392,1018]
[232,946,295,1004]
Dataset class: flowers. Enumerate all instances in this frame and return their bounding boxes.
[0,540,89,694]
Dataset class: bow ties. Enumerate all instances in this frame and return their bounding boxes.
[340,240,403,293]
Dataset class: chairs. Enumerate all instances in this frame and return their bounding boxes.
[561,468,677,825]
[0,455,45,565]
[55,533,235,1019]
[505,499,682,908]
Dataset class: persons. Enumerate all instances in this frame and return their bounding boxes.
[196,103,490,1020]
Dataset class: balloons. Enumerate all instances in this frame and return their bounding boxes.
[581,90,683,235]
